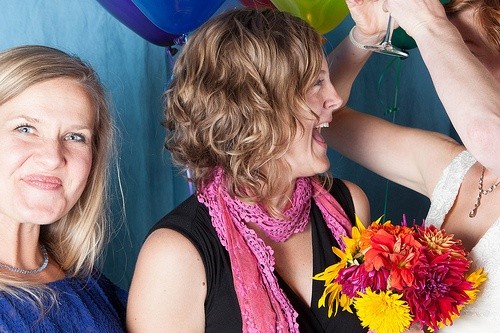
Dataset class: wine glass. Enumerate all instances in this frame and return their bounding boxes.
[364,13,408,58]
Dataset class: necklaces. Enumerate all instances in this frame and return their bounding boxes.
[0,241,51,276]
[470,164,500,230]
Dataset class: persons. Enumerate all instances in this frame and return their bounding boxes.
[0,44,127,333]
[127,9,424,333]
[319,0,499,333]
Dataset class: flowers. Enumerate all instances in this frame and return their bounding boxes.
[311,214,487,333]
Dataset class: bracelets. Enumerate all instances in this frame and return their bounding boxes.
[349,25,384,50]
